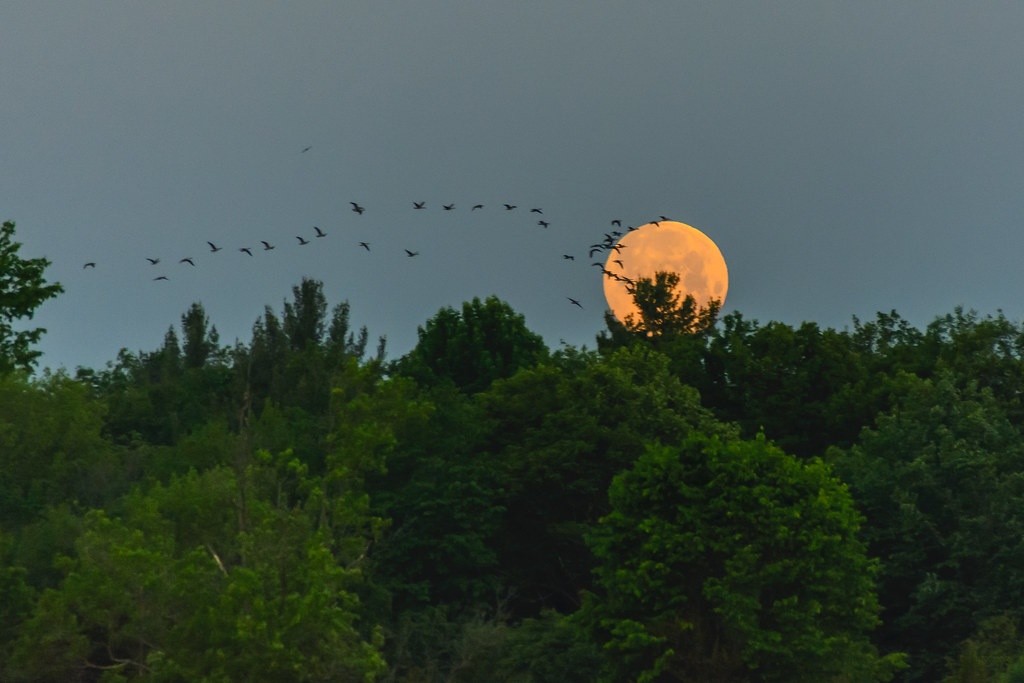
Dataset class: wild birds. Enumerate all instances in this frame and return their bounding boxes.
[153,276,168,281]
[240,248,253,257]
[537,220,549,228]
[145,257,160,265]
[179,258,196,267]
[314,226,327,238]
[301,145,312,153]
[358,242,371,248]
[412,201,427,210]
[562,214,670,310]
[502,204,516,211]
[84,263,96,270]
[530,208,543,215]
[442,203,455,211]
[207,241,222,253]
[471,205,485,212]
[260,240,275,250]
[404,248,420,257]
[295,236,310,246]
[349,202,365,215]
